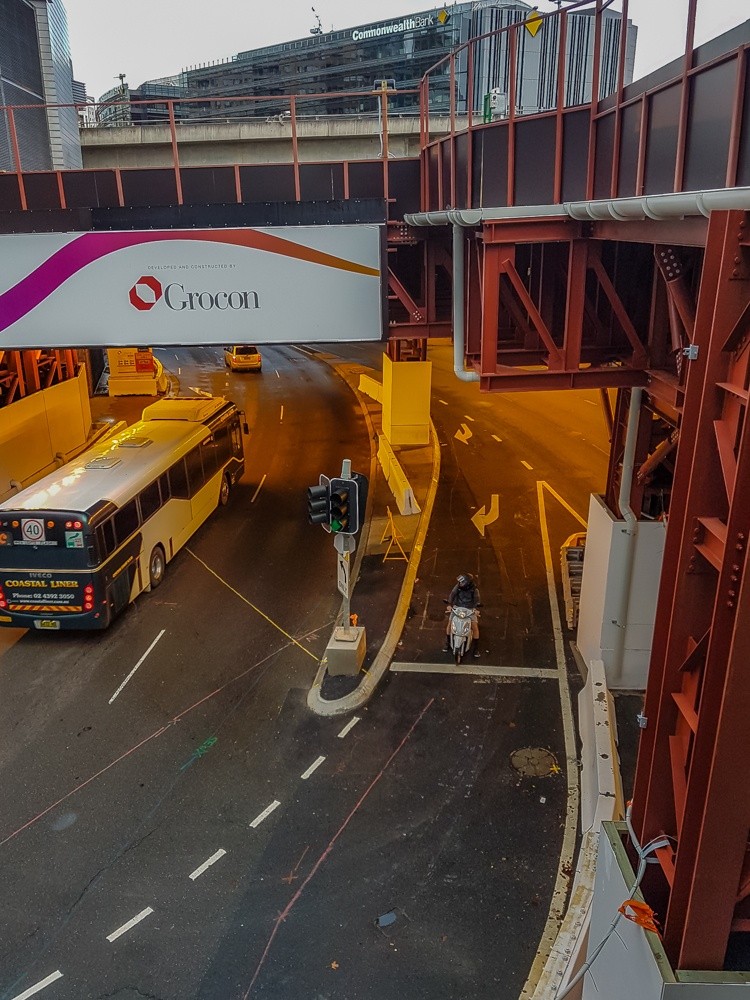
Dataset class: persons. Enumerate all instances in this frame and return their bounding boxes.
[443,574,482,657]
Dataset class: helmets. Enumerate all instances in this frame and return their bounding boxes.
[456,572,474,588]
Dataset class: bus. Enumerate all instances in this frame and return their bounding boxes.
[0,394,252,634]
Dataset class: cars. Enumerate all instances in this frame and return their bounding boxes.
[223,345,262,373]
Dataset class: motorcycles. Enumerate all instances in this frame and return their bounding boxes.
[442,598,485,665]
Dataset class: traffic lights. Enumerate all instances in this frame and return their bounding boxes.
[305,473,332,534]
[328,477,360,534]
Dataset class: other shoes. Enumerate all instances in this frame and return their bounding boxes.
[442,644,449,653]
[473,650,480,657]
